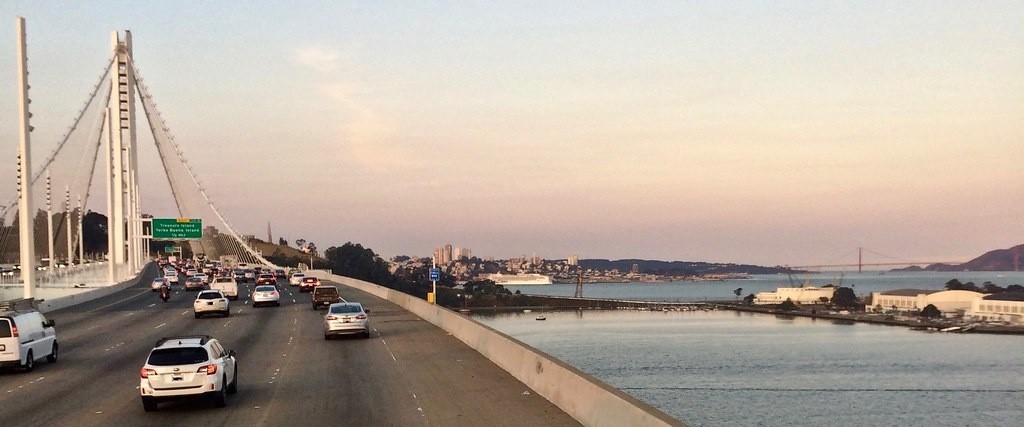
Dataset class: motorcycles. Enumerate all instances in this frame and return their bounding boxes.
[159,289,170,302]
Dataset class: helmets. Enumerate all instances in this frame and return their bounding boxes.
[162,281,166,285]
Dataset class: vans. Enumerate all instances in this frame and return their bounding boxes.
[0,310,58,371]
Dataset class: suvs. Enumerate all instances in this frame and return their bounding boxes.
[193,289,233,319]
[140,334,238,412]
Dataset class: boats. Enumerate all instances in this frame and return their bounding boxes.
[459,309,471,314]
[536,316,546,321]
[487,273,554,285]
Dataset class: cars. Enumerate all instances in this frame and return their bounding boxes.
[151,278,171,291]
[310,286,340,309]
[156,257,255,291]
[72,259,86,265]
[244,267,286,287]
[288,270,321,292]
[1,269,14,277]
[55,262,68,268]
[451,285,464,290]
[13,264,21,270]
[208,277,240,299]
[320,302,371,340]
[250,285,282,307]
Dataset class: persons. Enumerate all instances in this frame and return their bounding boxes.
[160,282,169,299]
